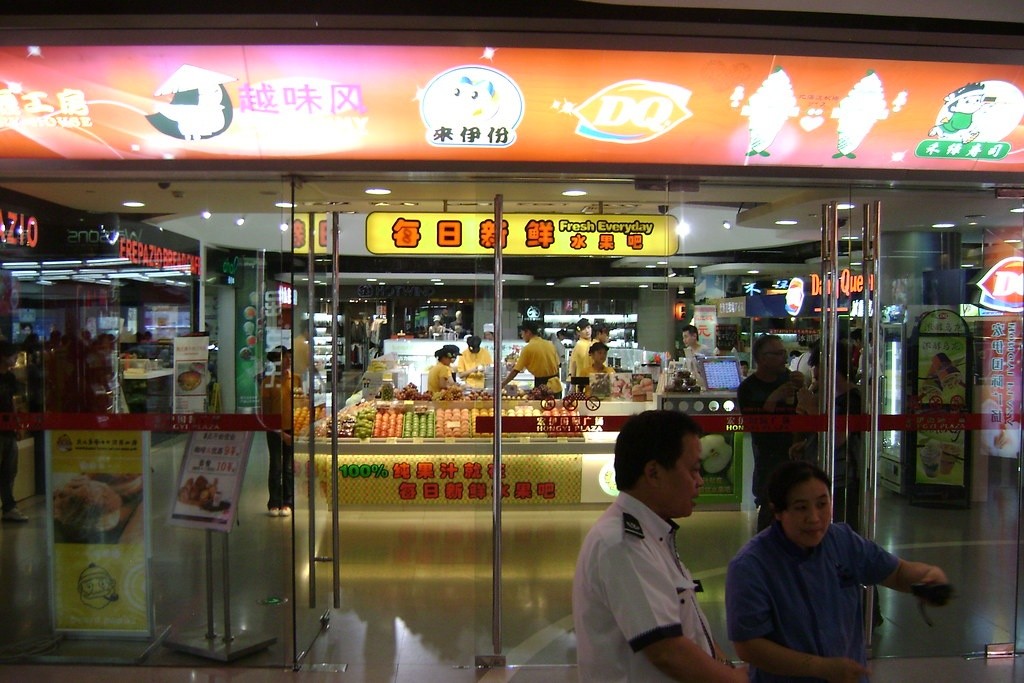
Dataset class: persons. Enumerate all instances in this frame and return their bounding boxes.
[429,315,446,340]
[260,345,302,517]
[502,318,632,404]
[0,328,157,522]
[427,324,494,404]
[572,408,750,683]
[449,311,471,339]
[725,461,949,683]
[681,324,883,631]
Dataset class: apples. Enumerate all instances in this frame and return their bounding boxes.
[353,408,436,437]
[500,406,577,416]
[434,408,469,437]
[698,433,733,474]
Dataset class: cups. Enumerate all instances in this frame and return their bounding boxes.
[940,444,961,475]
[919,449,941,478]
[922,367,943,397]
[932,353,962,390]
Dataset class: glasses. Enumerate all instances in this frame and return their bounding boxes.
[762,349,787,357]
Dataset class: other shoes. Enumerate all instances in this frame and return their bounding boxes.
[279,506,291,517]
[268,507,280,517]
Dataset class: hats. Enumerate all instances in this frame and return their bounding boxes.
[482,323,494,333]
[587,342,609,355]
[437,344,461,356]
[466,336,482,353]
[267,345,292,362]
[517,320,537,331]
[574,318,590,334]
[433,315,441,321]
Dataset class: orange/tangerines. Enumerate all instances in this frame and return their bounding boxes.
[471,407,496,436]
[294,407,327,437]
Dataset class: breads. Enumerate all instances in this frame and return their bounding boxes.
[52,470,141,532]
[178,361,205,390]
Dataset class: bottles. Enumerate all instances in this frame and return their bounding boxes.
[124,351,130,369]
[144,358,158,370]
[715,324,738,349]
[381,379,394,402]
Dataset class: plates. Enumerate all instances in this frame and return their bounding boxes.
[51,473,143,543]
[173,475,236,518]
